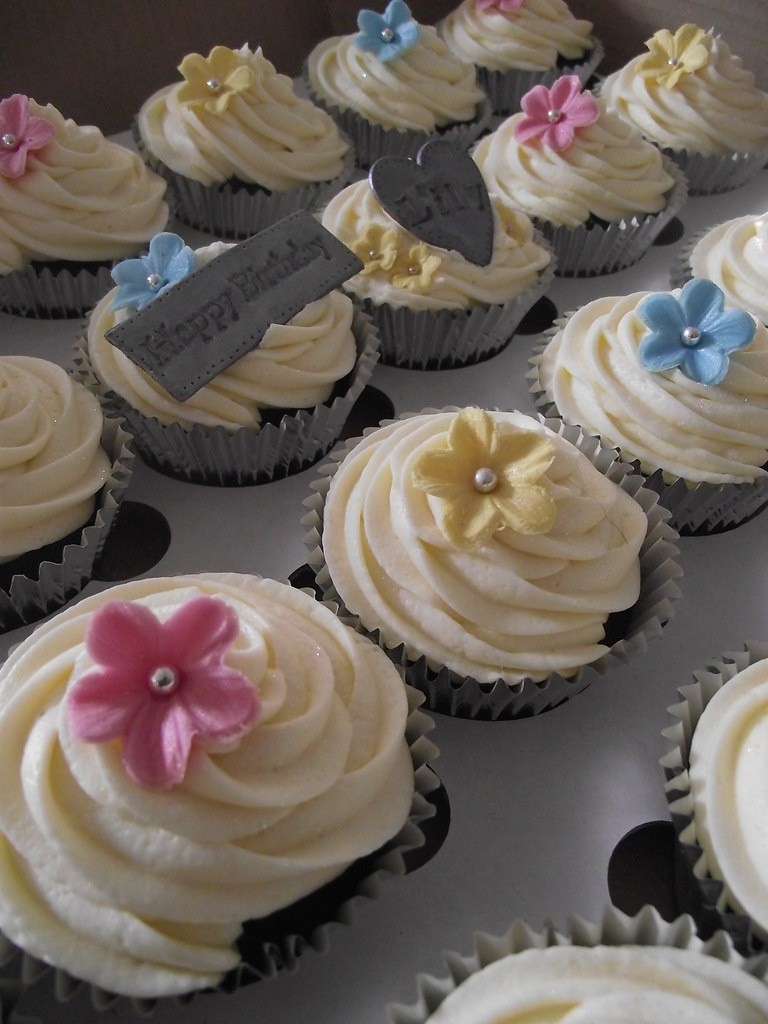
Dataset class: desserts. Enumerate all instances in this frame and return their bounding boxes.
[0,0,768,1024]
